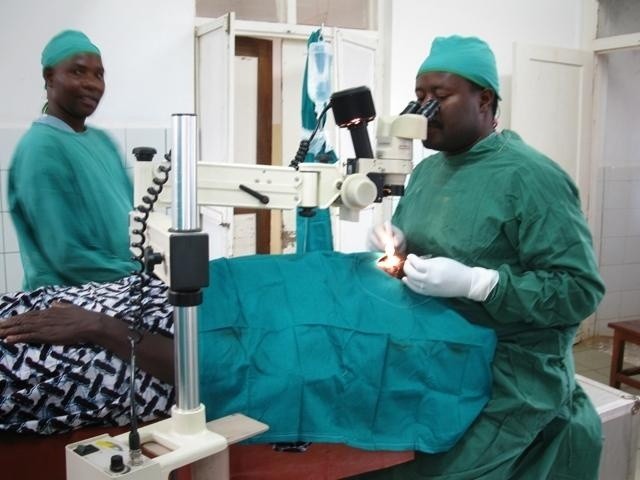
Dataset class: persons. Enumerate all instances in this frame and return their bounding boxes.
[367,35,606,480]
[0,249,497,455]
[7,30,145,290]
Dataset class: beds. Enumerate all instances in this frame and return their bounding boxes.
[0,423,415,480]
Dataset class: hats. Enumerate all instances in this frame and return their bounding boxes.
[417,34,500,100]
[41,30,101,68]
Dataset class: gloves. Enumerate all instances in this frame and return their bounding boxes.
[366,219,408,257]
[404,256,502,303]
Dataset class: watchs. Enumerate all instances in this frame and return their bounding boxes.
[125,329,142,343]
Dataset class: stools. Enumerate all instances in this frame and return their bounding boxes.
[608,319,640,395]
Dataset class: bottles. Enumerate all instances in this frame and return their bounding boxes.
[307,41,334,120]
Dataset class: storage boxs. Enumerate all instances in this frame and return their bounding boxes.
[572,374,640,479]
